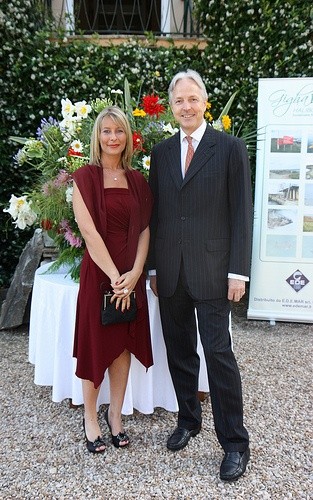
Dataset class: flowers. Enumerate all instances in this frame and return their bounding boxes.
[6,87,240,277]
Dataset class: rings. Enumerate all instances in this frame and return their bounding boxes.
[124,289,128,294]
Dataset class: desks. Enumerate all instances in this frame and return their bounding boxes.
[28,261,234,414]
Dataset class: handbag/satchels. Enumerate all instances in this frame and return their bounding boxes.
[101,291,137,325]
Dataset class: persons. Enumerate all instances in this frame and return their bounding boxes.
[71,105,154,453]
[148,68,254,480]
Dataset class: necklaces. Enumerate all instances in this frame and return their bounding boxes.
[114,177,117,181]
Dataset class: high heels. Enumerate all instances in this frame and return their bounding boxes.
[82,414,107,453]
[106,408,130,447]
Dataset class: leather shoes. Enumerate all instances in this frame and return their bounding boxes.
[166,425,201,451]
[220,447,250,479]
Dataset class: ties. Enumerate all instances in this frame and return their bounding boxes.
[185,136,194,175]
